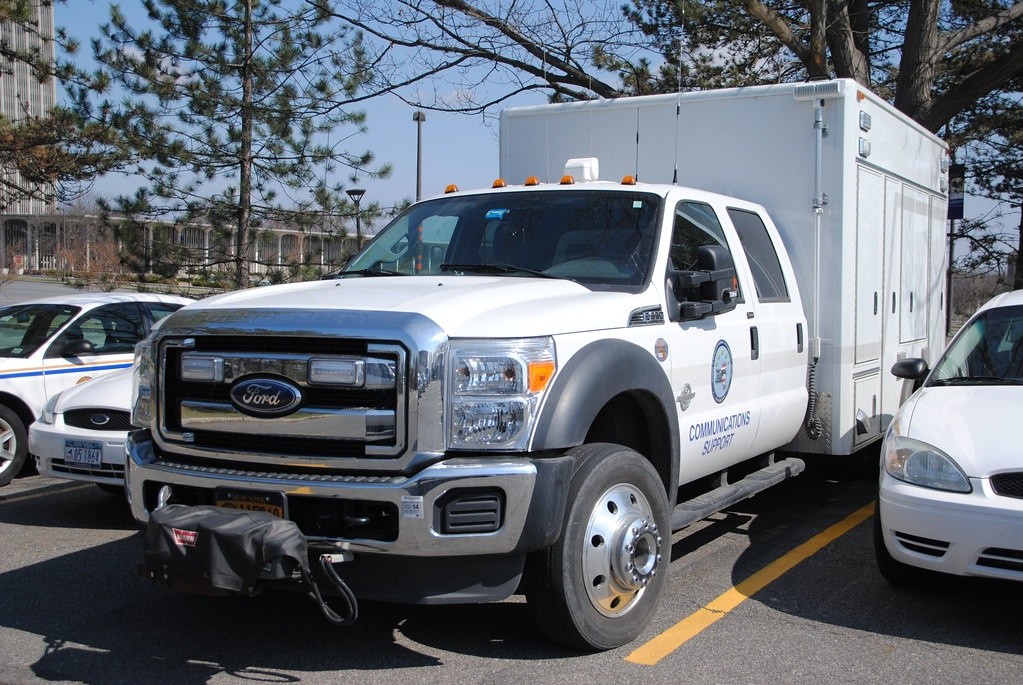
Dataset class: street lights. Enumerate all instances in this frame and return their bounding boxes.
[345,189,366,251]
[413,110,427,276]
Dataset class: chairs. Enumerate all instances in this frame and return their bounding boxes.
[57,324,85,354]
[103,310,143,346]
[493,220,551,268]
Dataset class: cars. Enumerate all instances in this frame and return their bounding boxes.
[0,288,199,484]
[871,285,1023,587]
[26,359,137,497]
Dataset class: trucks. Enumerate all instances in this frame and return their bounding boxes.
[123,76,954,655]
